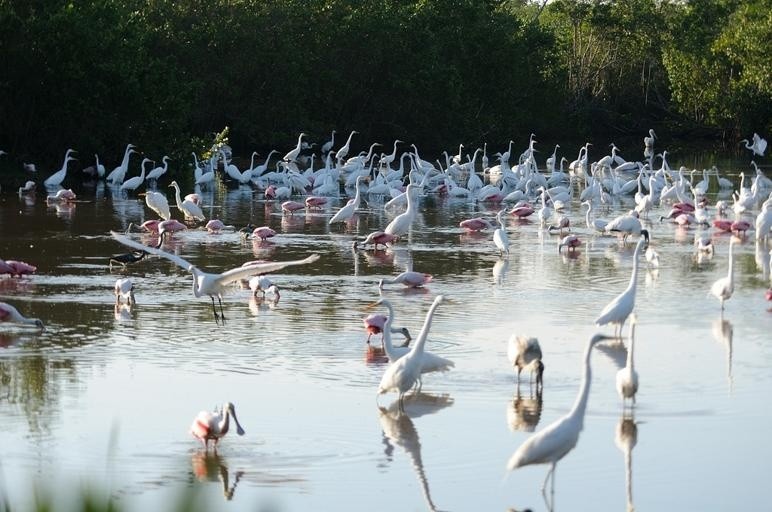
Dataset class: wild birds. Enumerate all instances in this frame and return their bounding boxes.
[191,455,244,501]
[44,127,772,334]
[614,405,640,512]
[371,288,456,415]
[712,310,736,384]
[376,399,442,512]
[19,195,37,207]
[505,314,641,489]
[19,161,38,195]
[1,254,43,332]
[190,401,246,454]
